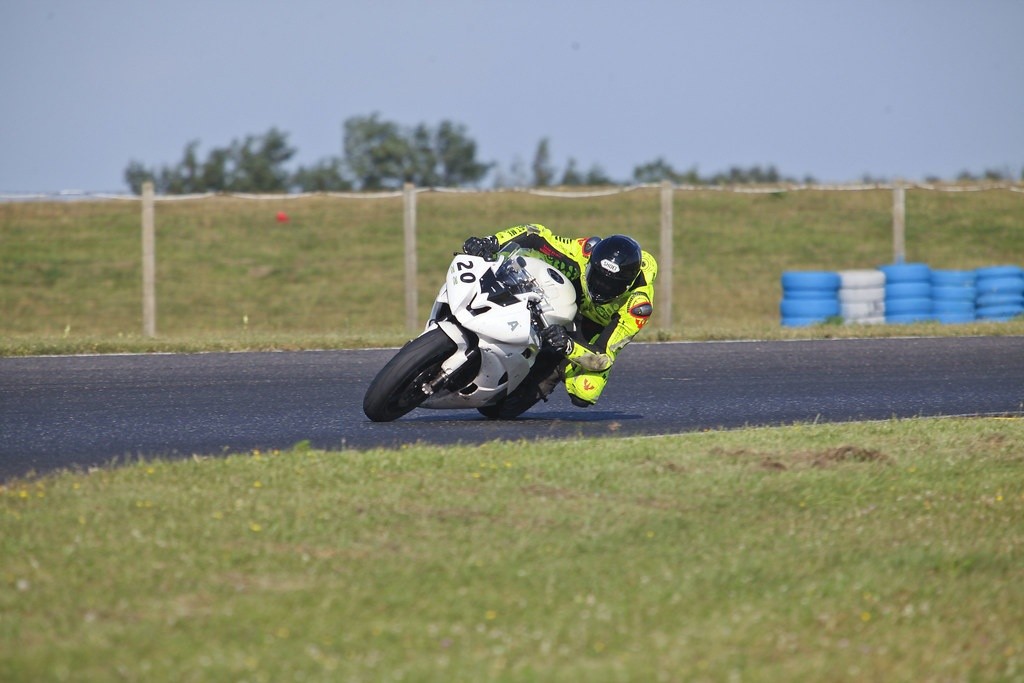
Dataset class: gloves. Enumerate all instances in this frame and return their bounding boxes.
[464,234,500,259]
[542,324,574,356]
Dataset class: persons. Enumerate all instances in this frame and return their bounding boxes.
[465,223,657,408]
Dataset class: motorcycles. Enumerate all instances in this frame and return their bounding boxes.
[363,238,578,423]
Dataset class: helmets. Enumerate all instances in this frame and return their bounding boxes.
[587,234,643,303]
